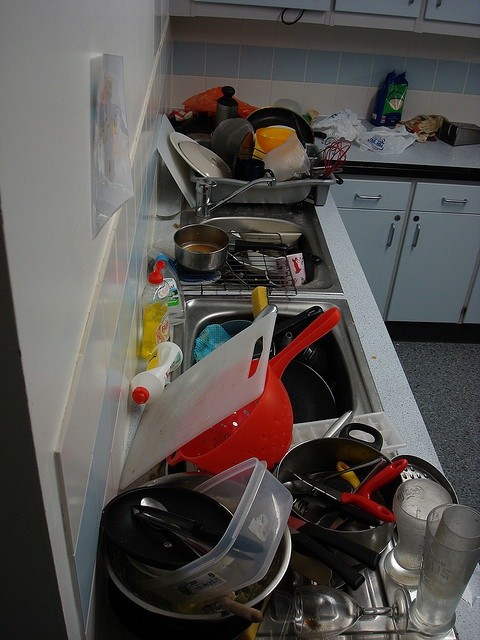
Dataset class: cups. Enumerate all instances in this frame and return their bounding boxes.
[409,505,480,635]
[383,480,451,588]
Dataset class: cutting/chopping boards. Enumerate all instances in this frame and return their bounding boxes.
[117,313,282,488]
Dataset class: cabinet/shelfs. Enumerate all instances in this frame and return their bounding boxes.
[330,163,480,335]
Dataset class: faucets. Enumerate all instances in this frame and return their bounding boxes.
[186,176,285,221]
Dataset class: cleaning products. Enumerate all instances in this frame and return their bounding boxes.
[140,253,169,362]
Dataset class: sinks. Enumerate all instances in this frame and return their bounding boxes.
[170,291,386,421]
[178,200,342,296]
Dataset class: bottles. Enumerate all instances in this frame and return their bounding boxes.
[217,86,239,121]
[154,253,186,327]
[131,342,184,405]
[135,259,168,366]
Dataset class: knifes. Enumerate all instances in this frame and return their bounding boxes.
[131,499,265,564]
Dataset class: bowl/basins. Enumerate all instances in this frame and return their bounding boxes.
[171,223,231,269]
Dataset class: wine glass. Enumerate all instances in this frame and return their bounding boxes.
[292,583,411,634]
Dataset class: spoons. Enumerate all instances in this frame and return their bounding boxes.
[140,496,225,585]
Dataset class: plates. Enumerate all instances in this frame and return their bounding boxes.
[166,130,212,177]
[178,140,231,180]
